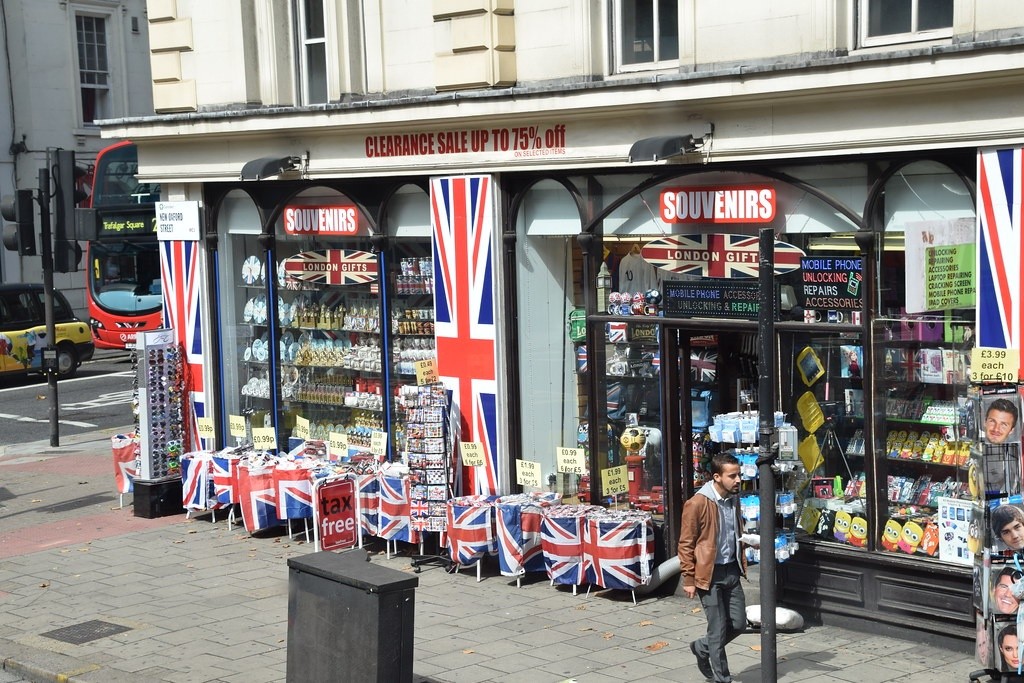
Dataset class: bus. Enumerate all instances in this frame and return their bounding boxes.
[85,140,161,349]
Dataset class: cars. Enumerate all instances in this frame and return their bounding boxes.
[0,282,95,380]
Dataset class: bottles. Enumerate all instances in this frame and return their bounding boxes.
[292,301,439,416]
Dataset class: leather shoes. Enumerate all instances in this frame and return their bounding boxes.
[714,677,739,683]
[689,640,715,680]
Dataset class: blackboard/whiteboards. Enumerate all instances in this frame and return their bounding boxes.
[663,280,777,321]
[800,256,863,311]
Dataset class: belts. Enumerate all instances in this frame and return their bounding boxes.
[715,560,738,568]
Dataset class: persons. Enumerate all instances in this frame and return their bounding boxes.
[998,624,1024,671]
[975,622,988,665]
[677,453,750,683]
[984,399,1018,444]
[994,567,1023,613]
[992,505,1024,549]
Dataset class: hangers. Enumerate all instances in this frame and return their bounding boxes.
[629,241,641,254]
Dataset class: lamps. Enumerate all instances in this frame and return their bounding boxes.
[807,232,905,251]
[238,150,310,183]
[626,122,716,164]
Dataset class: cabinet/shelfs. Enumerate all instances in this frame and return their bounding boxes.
[236,285,434,455]
[576,340,721,441]
[823,309,978,552]
